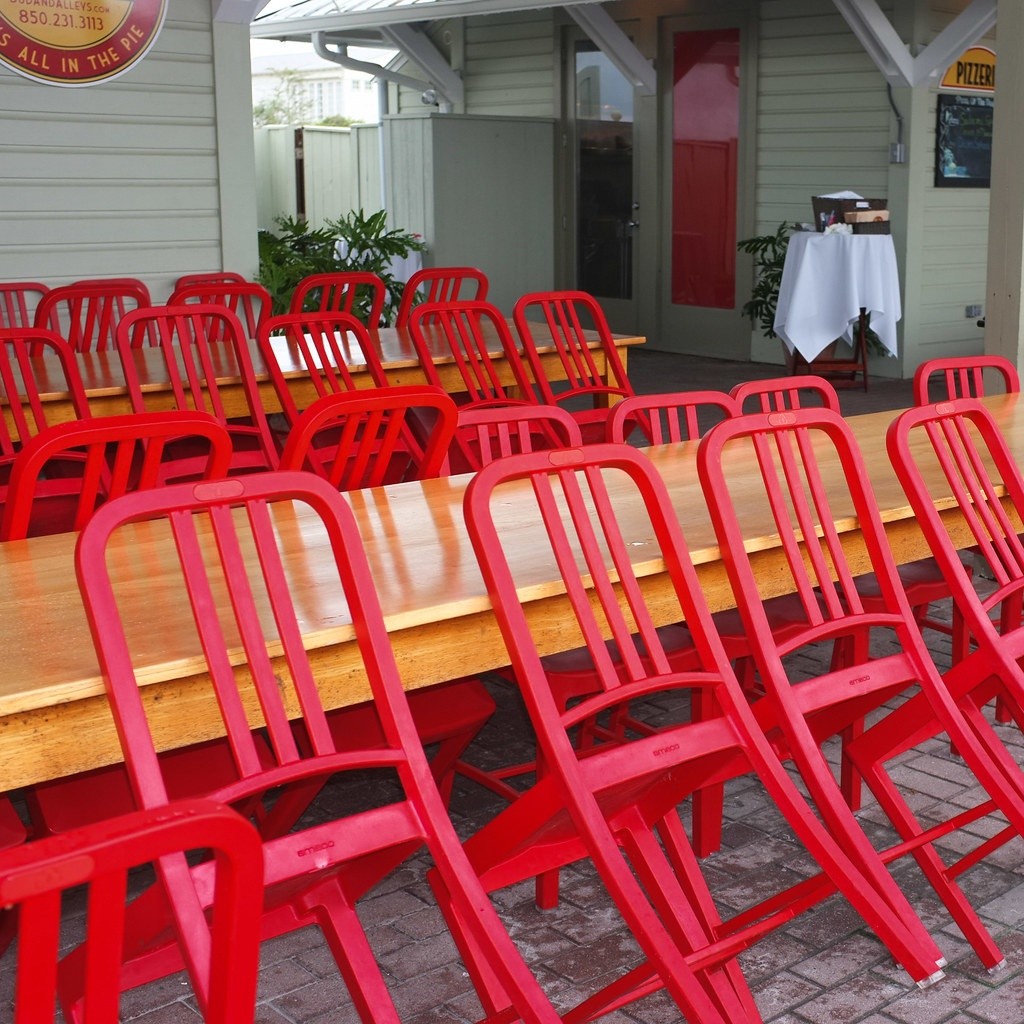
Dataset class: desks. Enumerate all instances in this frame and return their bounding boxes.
[765,233,903,393]
[0,382,1024,801]
[0,317,649,449]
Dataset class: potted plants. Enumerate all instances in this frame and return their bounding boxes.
[738,220,837,374]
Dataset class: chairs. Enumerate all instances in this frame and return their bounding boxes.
[0,261,1024,1024]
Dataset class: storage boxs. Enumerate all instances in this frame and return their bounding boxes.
[809,195,891,232]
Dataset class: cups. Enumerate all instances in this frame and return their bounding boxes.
[820,213,836,233]
